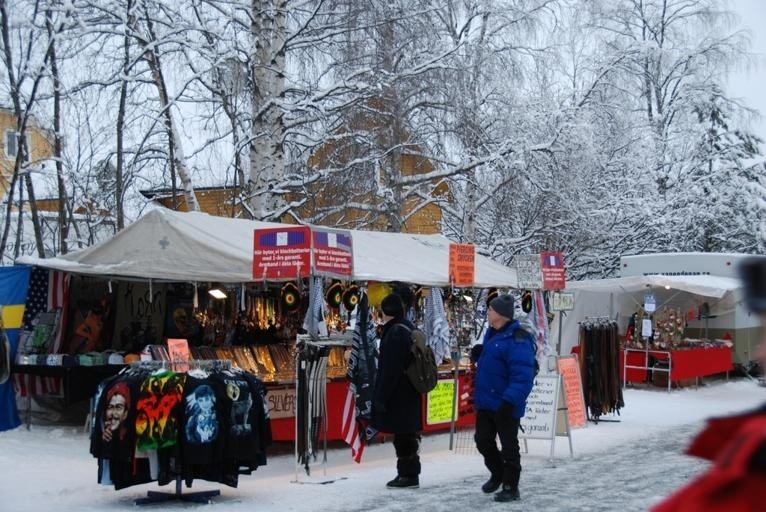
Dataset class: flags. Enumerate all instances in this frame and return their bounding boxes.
[11,267,70,397]
[1,266,30,434]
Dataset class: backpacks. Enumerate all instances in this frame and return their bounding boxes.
[384,320,437,393]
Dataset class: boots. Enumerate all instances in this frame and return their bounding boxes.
[482,450,503,493]
[493,463,522,502]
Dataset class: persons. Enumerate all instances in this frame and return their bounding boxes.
[471,294,540,500]
[370,294,424,489]
[102,382,130,444]
[183,384,220,441]
[648,254,766,511]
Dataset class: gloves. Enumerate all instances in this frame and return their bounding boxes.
[493,399,514,416]
[470,344,483,357]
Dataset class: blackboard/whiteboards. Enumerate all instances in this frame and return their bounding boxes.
[516,372,562,438]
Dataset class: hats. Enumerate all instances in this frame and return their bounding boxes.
[489,295,514,318]
[105,381,131,411]
[381,294,403,316]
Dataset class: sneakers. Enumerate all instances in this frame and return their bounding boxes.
[386,475,419,487]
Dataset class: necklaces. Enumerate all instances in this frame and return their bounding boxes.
[194,290,297,375]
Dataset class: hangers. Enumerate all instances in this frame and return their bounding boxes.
[120,358,241,380]
